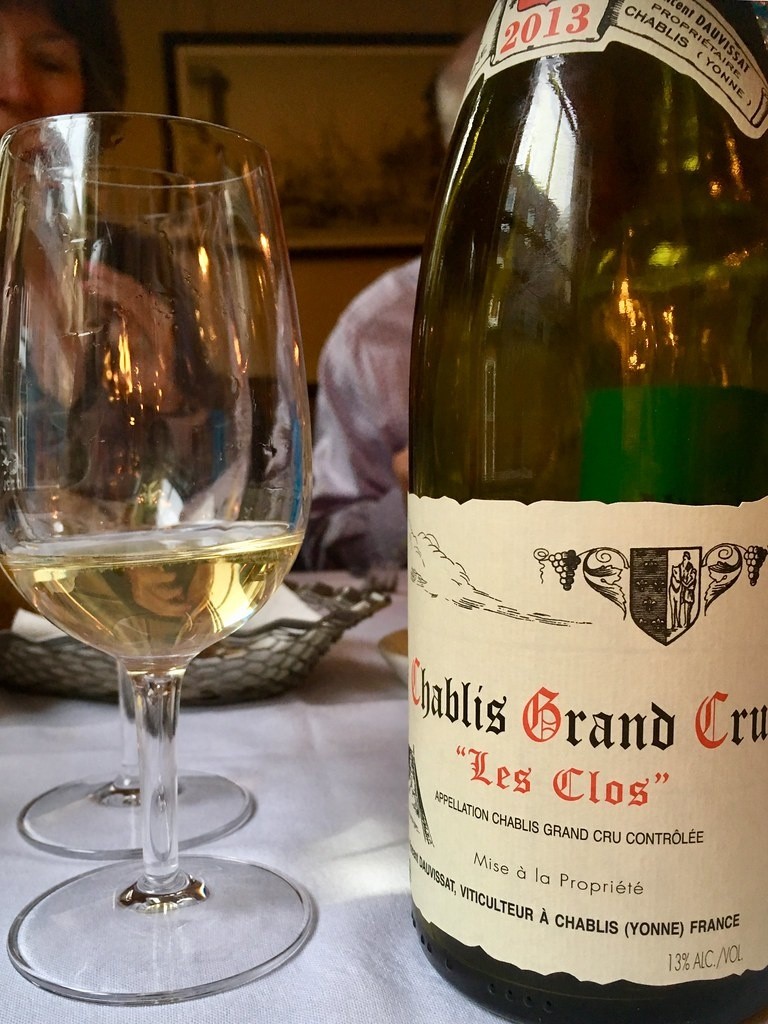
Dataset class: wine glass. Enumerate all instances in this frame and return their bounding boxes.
[2,109,319,1007]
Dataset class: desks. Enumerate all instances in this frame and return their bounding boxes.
[0,571,505,1024]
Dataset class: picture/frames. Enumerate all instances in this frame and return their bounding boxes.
[159,28,463,262]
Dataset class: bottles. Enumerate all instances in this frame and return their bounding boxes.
[403,1,768,1024]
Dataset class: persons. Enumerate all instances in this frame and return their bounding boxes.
[0,0,226,618]
[298,21,628,572]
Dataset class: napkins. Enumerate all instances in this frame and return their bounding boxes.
[10,585,329,695]
